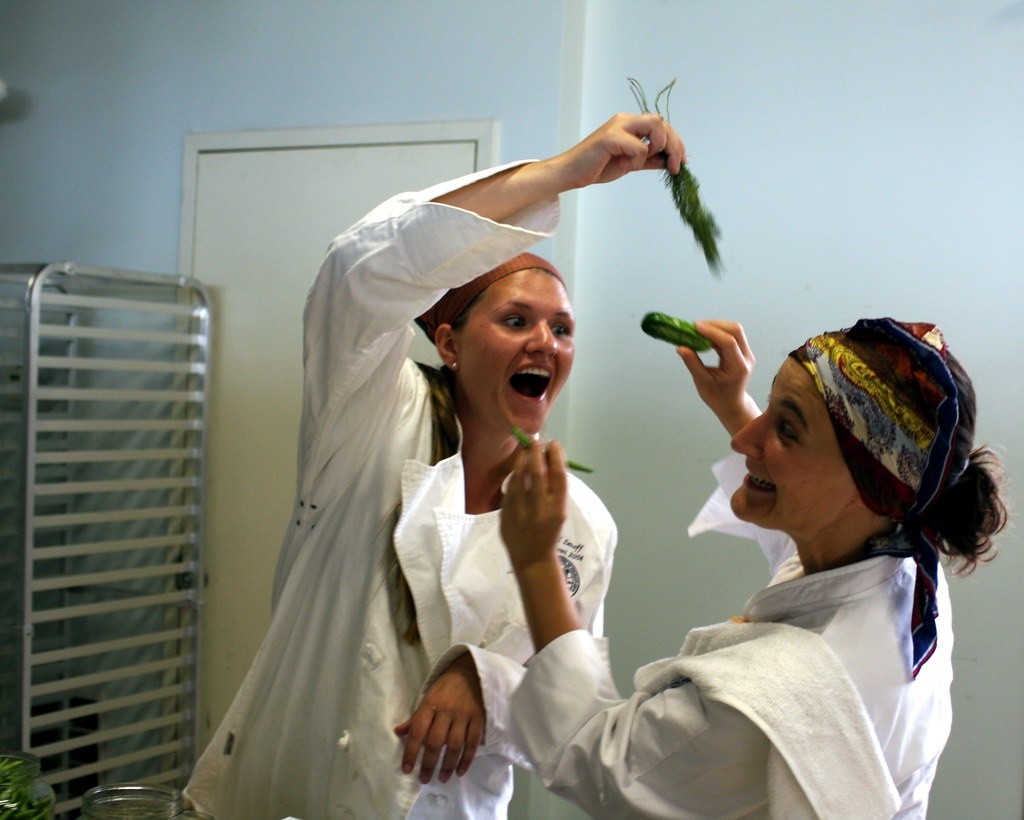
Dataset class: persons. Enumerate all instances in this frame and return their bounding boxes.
[180,110,689,820]
[498,313,1015,820]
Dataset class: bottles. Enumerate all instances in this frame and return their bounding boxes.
[0,750,56,820]
[76,784,215,820]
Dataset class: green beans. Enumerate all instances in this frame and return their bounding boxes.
[510,428,594,473]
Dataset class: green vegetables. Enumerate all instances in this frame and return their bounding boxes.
[626,76,724,269]
[0,756,55,820]
